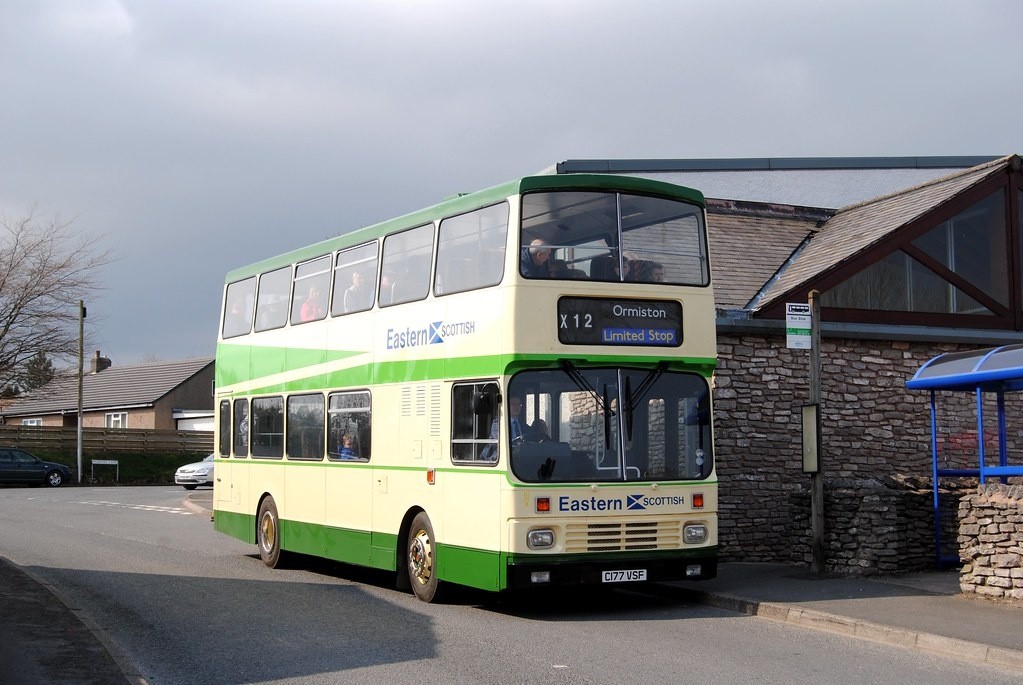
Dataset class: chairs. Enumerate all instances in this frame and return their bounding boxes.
[319,429,332,457]
[301,425,316,458]
[590,257,618,279]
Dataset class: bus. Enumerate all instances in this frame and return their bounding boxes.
[210,174,717,603]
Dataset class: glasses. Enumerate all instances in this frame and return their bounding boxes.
[511,404,523,408]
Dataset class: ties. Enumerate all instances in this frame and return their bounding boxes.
[513,421,521,438]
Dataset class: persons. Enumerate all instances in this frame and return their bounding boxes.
[479,396,547,460]
[613,256,630,279]
[241,405,260,446]
[648,263,667,283]
[521,239,550,279]
[342,433,360,459]
[301,287,322,321]
[349,272,364,310]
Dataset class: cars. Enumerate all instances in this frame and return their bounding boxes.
[175,453,214,490]
[0,446,71,488]
